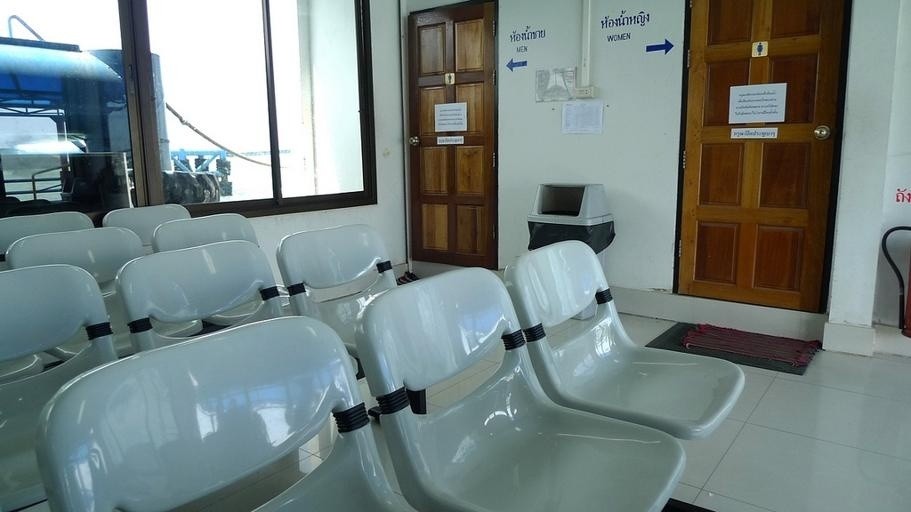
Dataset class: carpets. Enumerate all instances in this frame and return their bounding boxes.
[643,319,820,379]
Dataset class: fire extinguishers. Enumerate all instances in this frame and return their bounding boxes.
[882,226,911,338]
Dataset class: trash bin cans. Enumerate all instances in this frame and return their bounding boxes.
[527,182,616,320]
